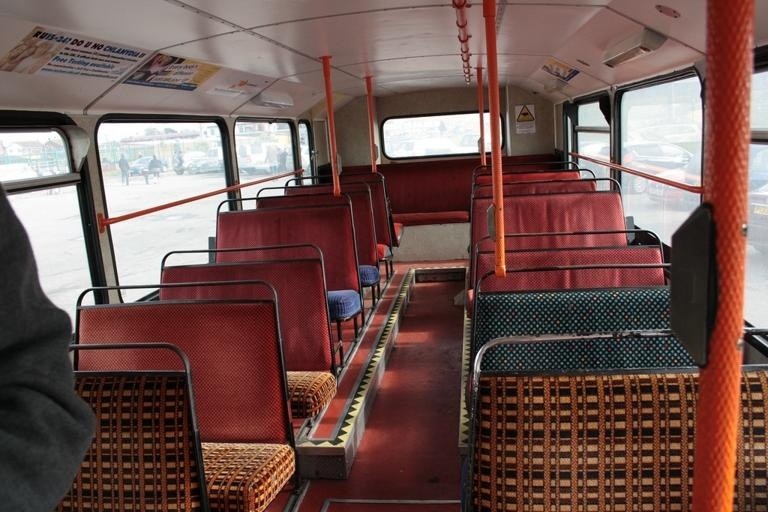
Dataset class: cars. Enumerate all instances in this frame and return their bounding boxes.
[582,143,692,196]
[646,141,767,254]
[130,157,164,174]
[188,158,225,175]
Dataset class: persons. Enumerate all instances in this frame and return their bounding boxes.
[0,184,97,510]
[118,155,130,186]
[264,140,281,182]
[148,153,165,185]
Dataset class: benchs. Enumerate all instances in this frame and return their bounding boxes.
[311,156,567,262]
[57,161,401,512]
[462,156,767,507]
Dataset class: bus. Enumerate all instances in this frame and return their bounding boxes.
[210,134,261,163]
